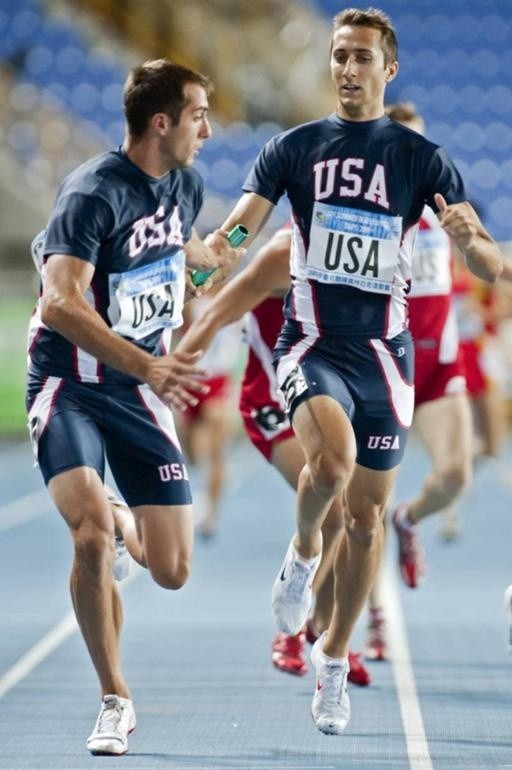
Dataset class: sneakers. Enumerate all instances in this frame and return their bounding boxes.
[272,530,321,636]
[269,609,386,686]
[84,696,138,754]
[103,486,136,582]
[310,634,354,738]
[395,504,425,591]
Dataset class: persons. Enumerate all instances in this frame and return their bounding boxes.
[23,55,247,757]
[166,9,511,737]
